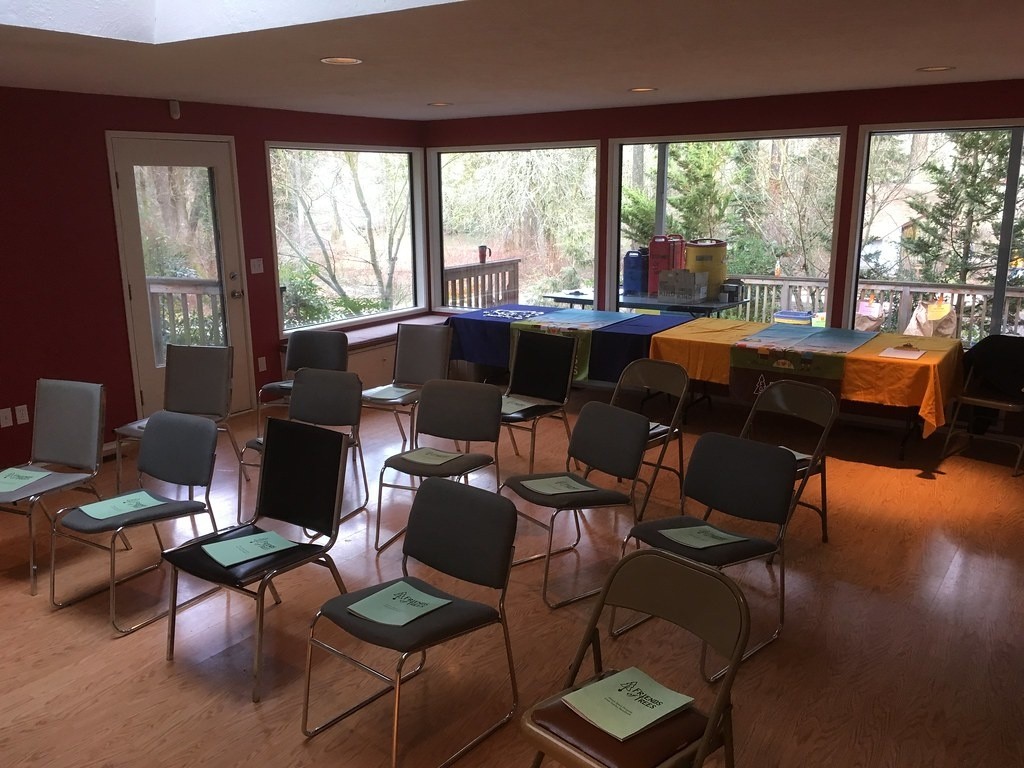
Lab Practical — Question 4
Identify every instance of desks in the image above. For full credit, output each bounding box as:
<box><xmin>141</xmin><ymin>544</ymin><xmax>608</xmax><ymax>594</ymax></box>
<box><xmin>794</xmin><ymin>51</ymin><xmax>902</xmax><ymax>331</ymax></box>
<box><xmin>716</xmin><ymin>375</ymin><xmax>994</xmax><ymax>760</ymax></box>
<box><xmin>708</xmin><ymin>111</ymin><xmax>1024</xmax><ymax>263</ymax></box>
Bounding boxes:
<box><xmin>545</xmin><ymin>286</ymin><xmax>751</xmax><ymax>318</ymax></box>
<box><xmin>447</xmin><ymin>303</ymin><xmax>963</xmax><ymax>479</ymax></box>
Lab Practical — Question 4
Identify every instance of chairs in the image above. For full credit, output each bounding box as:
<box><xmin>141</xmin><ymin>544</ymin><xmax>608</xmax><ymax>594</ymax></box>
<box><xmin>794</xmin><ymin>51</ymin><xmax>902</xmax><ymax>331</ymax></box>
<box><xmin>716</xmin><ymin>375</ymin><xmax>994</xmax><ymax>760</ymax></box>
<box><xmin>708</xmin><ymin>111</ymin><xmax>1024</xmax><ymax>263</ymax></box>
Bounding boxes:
<box><xmin>0</xmin><ymin>321</ymin><xmax>841</xmax><ymax>768</ymax></box>
<box><xmin>934</xmin><ymin>335</ymin><xmax>1024</xmax><ymax>477</ymax></box>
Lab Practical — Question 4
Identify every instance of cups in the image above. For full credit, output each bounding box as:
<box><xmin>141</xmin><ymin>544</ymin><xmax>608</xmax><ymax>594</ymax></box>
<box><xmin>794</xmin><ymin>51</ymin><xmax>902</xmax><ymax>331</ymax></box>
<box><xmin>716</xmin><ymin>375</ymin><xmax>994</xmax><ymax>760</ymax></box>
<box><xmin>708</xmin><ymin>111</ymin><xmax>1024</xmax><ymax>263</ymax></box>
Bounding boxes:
<box><xmin>479</xmin><ymin>246</ymin><xmax>491</xmax><ymax>263</ymax></box>
<box><xmin>623</xmin><ymin>247</ymin><xmax>648</xmax><ymax>297</ymax></box>
<box><xmin>718</xmin><ymin>293</ymin><xmax>728</xmax><ymax>302</ymax></box>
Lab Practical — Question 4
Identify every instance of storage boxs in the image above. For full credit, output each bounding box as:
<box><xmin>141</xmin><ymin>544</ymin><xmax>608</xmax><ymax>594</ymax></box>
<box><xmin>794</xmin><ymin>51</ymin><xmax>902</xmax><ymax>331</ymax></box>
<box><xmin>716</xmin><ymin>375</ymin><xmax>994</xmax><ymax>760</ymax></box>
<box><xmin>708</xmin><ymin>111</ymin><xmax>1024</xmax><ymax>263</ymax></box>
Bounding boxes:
<box><xmin>720</xmin><ymin>278</ymin><xmax>745</xmax><ymax>301</ymax></box>
<box><xmin>657</xmin><ymin>268</ymin><xmax>709</xmax><ymax>304</ymax></box>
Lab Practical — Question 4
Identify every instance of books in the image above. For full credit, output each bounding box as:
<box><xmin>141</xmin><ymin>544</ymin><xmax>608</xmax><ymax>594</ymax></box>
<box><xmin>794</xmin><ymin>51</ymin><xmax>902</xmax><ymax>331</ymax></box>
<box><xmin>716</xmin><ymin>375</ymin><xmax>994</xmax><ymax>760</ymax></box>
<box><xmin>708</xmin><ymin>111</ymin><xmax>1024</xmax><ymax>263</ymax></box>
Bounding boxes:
<box><xmin>77</xmin><ymin>490</ymin><xmax>168</xmax><ymax>521</ymax></box>
<box><xmin>366</xmin><ymin>386</ymin><xmax>416</xmax><ymax>400</ymax></box>
<box><xmin>561</xmin><ymin>666</ymin><xmax>695</xmax><ymax>741</ymax></box>
<box><xmin>657</xmin><ymin>525</ymin><xmax>750</xmax><ymax>548</ymax></box>
<box><xmin>0</xmin><ymin>467</ymin><xmax>53</xmax><ymax>493</ymax></box>
<box><xmin>401</xmin><ymin>447</ymin><xmax>463</xmax><ymax>466</ymax></box>
<box><xmin>346</xmin><ymin>580</ymin><xmax>452</xmax><ymax>627</ymax></box>
<box><xmin>519</xmin><ymin>475</ymin><xmax>599</xmax><ymax>496</ymax></box>
<box><xmin>200</xmin><ymin>530</ymin><xmax>299</xmax><ymax>568</ymax></box>
<box><xmin>501</xmin><ymin>396</ymin><xmax>538</xmax><ymax>415</ymax></box>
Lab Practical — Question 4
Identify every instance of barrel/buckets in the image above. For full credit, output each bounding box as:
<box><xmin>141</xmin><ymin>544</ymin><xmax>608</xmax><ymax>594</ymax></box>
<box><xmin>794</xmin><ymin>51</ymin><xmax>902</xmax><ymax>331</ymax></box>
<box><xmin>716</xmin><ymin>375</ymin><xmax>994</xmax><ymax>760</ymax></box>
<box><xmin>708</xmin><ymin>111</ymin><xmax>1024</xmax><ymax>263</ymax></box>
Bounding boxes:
<box><xmin>684</xmin><ymin>238</ymin><xmax>727</xmax><ymax>300</ymax></box>
<box><xmin>648</xmin><ymin>234</ymin><xmax>685</xmax><ymax>297</ymax></box>
